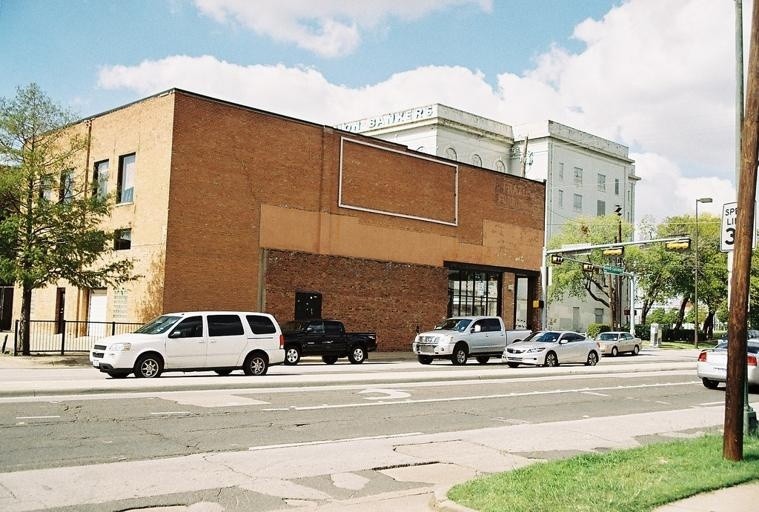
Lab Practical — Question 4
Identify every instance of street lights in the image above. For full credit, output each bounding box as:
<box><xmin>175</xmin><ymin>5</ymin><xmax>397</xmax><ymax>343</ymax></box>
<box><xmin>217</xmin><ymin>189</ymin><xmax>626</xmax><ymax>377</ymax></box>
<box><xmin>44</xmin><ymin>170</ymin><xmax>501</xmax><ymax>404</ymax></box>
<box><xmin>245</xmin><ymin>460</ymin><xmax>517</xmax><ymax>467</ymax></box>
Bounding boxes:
<box><xmin>695</xmin><ymin>197</ymin><xmax>713</xmax><ymax>348</ymax></box>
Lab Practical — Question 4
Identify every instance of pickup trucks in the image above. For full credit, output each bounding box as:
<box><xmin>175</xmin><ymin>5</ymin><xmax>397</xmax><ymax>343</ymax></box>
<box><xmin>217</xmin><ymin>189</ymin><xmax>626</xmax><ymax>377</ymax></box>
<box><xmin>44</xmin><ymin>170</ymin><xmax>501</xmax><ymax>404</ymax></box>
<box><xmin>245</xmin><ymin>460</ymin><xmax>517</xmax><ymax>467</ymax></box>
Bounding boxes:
<box><xmin>412</xmin><ymin>316</ymin><xmax>533</xmax><ymax>365</ymax></box>
<box><xmin>280</xmin><ymin>319</ymin><xmax>376</xmax><ymax>366</ymax></box>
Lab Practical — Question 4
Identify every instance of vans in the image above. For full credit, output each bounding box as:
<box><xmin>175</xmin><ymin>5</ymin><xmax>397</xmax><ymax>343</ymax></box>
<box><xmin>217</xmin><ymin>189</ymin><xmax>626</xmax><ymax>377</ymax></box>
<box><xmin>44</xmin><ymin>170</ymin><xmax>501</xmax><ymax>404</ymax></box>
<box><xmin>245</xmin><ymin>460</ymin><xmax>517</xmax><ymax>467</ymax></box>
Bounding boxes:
<box><xmin>90</xmin><ymin>312</ymin><xmax>286</xmax><ymax>378</ymax></box>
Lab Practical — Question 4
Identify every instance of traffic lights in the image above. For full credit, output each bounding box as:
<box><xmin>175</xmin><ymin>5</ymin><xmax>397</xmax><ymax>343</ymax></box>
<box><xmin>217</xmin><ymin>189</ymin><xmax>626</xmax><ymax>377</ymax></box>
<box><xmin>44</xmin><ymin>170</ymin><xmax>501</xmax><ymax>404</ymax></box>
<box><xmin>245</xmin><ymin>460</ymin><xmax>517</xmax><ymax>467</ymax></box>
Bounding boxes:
<box><xmin>602</xmin><ymin>247</ymin><xmax>624</xmax><ymax>257</ymax></box>
<box><xmin>581</xmin><ymin>263</ymin><xmax>595</xmax><ymax>272</ymax></box>
<box><xmin>665</xmin><ymin>239</ymin><xmax>691</xmax><ymax>253</ymax></box>
<box><xmin>550</xmin><ymin>255</ymin><xmax>564</xmax><ymax>265</ymax></box>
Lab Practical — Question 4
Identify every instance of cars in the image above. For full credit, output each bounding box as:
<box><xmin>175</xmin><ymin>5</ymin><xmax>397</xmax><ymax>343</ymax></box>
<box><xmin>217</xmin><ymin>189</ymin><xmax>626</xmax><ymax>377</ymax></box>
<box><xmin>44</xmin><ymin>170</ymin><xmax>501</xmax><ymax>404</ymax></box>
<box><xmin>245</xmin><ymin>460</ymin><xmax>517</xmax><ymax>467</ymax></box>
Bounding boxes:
<box><xmin>501</xmin><ymin>331</ymin><xmax>601</xmax><ymax>368</ymax></box>
<box><xmin>697</xmin><ymin>329</ymin><xmax>759</xmax><ymax>388</ymax></box>
<box><xmin>594</xmin><ymin>332</ymin><xmax>643</xmax><ymax>356</ymax></box>
